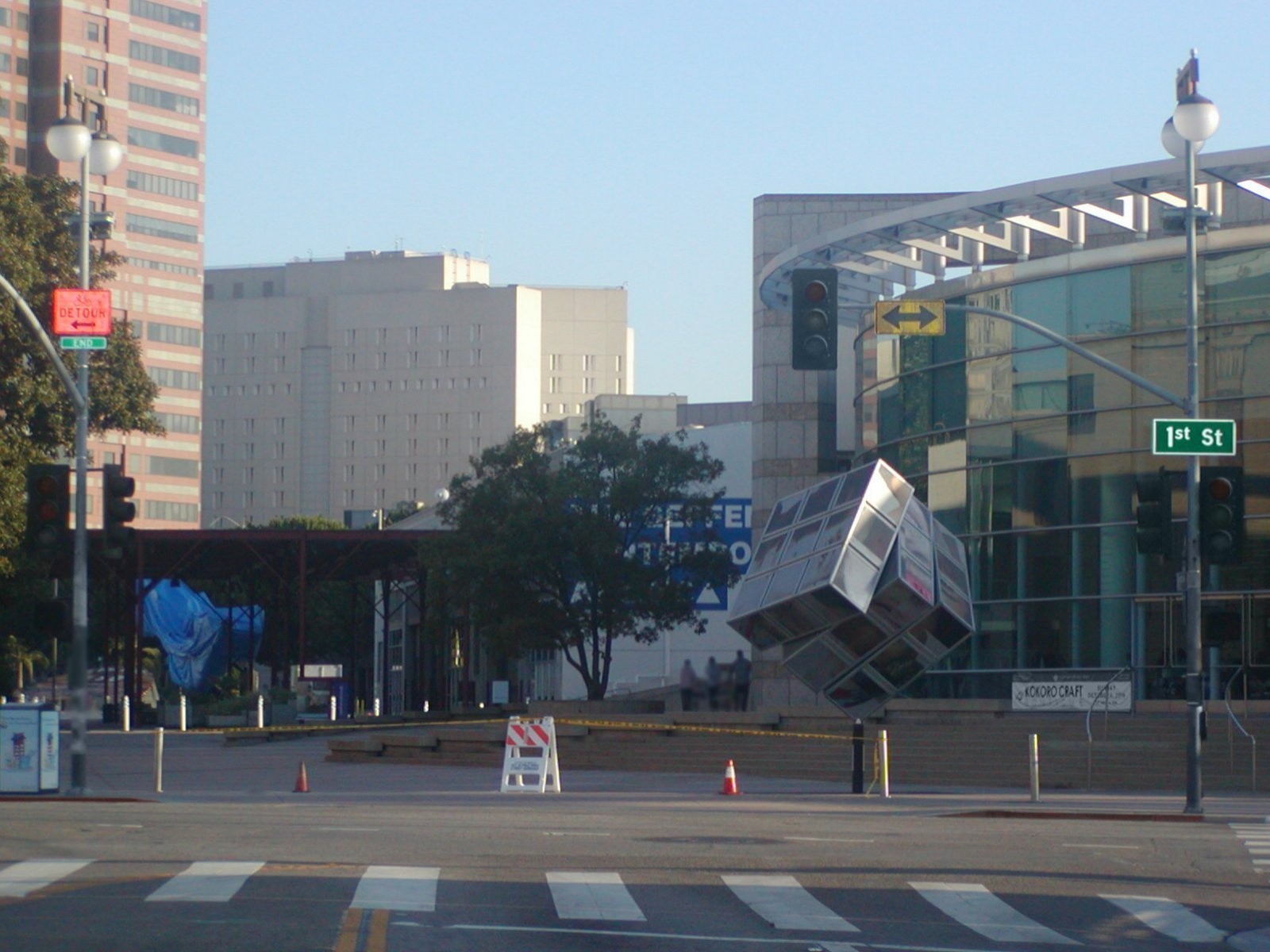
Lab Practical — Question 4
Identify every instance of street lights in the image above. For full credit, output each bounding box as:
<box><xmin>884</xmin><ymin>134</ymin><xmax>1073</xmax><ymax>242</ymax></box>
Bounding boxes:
<box><xmin>1159</xmin><ymin>46</ymin><xmax>1221</xmax><ymax>816</ymax></box>
<box><xmin>43</xmin><ymin>74</ymin><xmax>125</xmax><ymax>798</ymax></box>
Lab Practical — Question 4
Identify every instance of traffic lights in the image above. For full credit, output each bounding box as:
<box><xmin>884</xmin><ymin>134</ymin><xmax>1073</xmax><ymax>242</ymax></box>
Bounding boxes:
<box><xmin>1136</xmin><ymin>474</ymin><xmax>1173</xmax><ymax>561</ymax></box>
<box><xmin>1198</xmin><ymin>464</ymin><xmax>1246</xmax><ymax>560</ymax></box>
<box><xmin>103</xmin><ymin>462</ymin><xmax>136</xmax><ymax>561</ymax></box>
<box><xmin>792</xmin><ymin>267</ymin><xmax>839</xmax><ymax>371</ymax></box>
<box><xmin>25</xmin><ymin>463</ymin><xmax>71</xmax><ymax>563</ymax></box>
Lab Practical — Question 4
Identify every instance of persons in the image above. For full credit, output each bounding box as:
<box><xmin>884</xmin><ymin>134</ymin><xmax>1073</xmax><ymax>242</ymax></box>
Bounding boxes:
<box><xmin>680</xmin><ymin>659</ymin><xmax>696</xmax><ymax>711</ymax></box>
<box><xmin>728</xmin><ymin>649</ymin><xmax>751</xmax><ymax>711</ymax></box>
<box><xmin>703</xmin><ymin>656</ymin><xmax>720</xmax><ymax>710</ymax></box>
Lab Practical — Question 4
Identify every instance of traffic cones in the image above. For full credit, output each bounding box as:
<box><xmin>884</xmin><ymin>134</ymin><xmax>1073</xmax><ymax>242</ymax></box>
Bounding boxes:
<box><xmin>292</xmin><ymin>761</ymin><xmax>312</xmax><ymax>793</ymax></box>
<box><xmin>718</xmin><ymin>759</ymin><xmax>743</xmax><ymax>796</ymax></box>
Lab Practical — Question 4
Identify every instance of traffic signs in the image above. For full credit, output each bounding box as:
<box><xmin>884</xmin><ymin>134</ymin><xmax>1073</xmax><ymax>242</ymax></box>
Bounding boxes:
<box><xmin>51</xmin><ymin>290</ymin><xmax>113</xmax><ymax>338</ymax></box>
